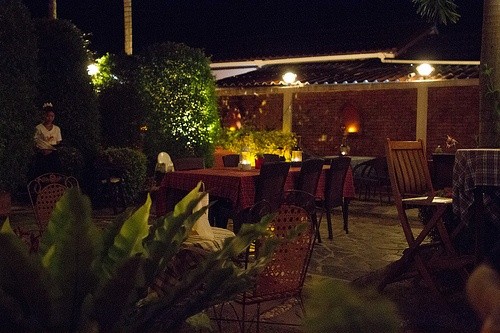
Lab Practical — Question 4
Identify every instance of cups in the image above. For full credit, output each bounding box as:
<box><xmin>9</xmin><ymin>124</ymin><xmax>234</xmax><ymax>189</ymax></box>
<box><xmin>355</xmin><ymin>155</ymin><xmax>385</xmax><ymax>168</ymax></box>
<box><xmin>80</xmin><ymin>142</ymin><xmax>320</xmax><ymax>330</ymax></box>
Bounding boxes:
<box><xmin>241</xmin><ymin>151</ymin><xmax>251</xmax><ymax>171</ymax></box>
<box><xmin>291</xmin><ymin>150</ymin><xmax>302</xmax><ymax>162</ymax></box>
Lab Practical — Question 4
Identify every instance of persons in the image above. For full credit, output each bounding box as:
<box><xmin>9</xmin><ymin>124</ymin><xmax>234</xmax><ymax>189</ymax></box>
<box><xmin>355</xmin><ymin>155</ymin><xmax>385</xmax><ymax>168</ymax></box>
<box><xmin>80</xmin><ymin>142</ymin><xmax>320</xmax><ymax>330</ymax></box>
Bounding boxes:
<box><xmin>32</xmin><ymin>103</ymin><xmax>62</xmax><ymax>179</ymax></box>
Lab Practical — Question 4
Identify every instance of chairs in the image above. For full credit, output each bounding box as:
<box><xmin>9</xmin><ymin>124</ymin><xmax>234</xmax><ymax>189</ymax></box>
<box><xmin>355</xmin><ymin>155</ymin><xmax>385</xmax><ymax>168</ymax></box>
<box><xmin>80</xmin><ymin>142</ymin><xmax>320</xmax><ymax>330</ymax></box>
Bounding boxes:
<box><xmin>153</xmin><ymin>152</ymin><xmax>392</xmax><ymax>333</ymax></box>
<box><xmin>27</xmin><ymin>171</ymin><xmax>80</xmax><ymax>240</ymax></box>
<box><xmin>377</xmin><ymin>138</ymin><xmax>460</xmax><ymax>301</ymax></box>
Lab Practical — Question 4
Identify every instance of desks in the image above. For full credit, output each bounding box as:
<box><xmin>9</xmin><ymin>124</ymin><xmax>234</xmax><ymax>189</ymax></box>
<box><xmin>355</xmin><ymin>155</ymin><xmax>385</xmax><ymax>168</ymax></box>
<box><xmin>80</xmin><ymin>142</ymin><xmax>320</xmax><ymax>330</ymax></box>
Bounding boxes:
<box><xmin>324</xmin><ymin>156</ymin><xmax>376</xmax><ymax>190</ymax></box>
<box><xmin>181</xmin><ymin>223</ymin><xmax>236</xmax><ymax>251</ymax></box>
<box><xmin>431</xmin><ymin>152</ymin><xmax>454</xmax><ymax>186</ymax></box>
<box><xmin>452</xmin><ymin>149</ymin><xmax>500</xmax><ymax>268</ymax></box>
<box><xmin>157</xmin><ymin>165</ymin><xmax>356</xmax><ymax>239</ymax></box>
